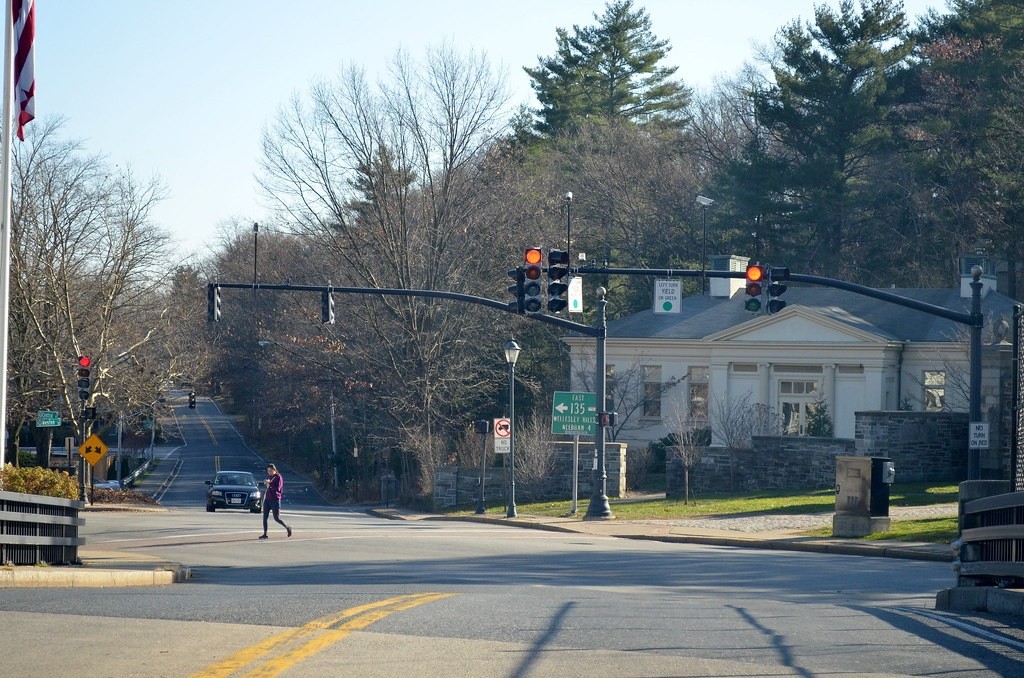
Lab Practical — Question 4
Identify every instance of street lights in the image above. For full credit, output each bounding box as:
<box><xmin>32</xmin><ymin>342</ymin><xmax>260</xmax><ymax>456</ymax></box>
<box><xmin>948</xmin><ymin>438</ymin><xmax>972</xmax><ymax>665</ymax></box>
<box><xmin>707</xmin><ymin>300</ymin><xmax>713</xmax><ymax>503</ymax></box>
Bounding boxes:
<box><xmin>502</xmin><ymin>335</ymin><xmax>521</xmax><ymax>518</ymax></box>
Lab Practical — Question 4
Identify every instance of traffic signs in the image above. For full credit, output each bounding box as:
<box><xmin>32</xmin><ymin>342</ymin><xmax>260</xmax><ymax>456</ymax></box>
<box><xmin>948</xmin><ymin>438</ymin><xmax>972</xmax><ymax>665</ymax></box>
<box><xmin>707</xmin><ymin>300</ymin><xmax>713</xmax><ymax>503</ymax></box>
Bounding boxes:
<box><xmin>552</xmin><ymin>391</ymin><xmax>596</xmax><ymax>435</ymax></box>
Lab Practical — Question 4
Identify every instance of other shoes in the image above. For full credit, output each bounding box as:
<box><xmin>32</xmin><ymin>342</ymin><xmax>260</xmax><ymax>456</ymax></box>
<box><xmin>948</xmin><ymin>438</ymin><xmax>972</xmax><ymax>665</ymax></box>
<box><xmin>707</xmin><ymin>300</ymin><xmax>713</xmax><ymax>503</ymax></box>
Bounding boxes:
<box><xmin>259</xmin><ymin>535</ymin><xmax>268</xmax><ymax>539</ymax></box>
<box><xmin>287</xmin><ymin>526</ymin><xmax>292</xmax><ymax>537</ymax></box>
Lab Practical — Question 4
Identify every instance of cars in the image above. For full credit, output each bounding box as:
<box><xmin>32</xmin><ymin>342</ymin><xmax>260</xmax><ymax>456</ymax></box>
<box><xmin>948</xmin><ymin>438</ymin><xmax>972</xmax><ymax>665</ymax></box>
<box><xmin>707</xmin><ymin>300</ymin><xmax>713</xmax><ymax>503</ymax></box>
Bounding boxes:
<box><xmin>205</xmin><ymin>471</ymin><xmax>266</xmax><ymax>514</ymax></box>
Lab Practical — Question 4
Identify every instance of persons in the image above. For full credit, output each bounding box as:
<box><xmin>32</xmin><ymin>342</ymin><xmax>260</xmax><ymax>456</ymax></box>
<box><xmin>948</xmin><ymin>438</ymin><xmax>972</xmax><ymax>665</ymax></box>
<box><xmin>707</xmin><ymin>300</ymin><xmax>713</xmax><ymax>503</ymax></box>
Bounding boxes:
<box><xmin>188</xmin><ymin>385</ymin><xmax>197</xmax><ymax>406</ymax></box>
<box><xmin>221</xmin><ymin>476</ymin><xmax>228</xmax><ymax>485</ymax></box>
<box><xmin>239</xmin><ymin>476</ymin><xmax>251</xmax><ymax>485</ymax></box>
<box><xmin>259</xmin><ymin>463</ymin><xmax>292</xmax><ymax>540</ymax></box>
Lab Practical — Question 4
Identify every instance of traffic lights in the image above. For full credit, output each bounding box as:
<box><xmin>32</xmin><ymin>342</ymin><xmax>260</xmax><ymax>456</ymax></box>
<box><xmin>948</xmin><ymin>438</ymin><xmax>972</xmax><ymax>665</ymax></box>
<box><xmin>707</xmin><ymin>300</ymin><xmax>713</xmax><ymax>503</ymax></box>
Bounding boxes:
<box><xmin>598</xmin><ymin>412</ymin><xmax>617</xmax><ymax>426</ymax></box>
<box><xmin>86</xmin><ymin>407</ymin><xmax>96</xmax><ymax>419</ymax></box>
<box><xmin>745</xmin><ymin>265</ymin><xmax>763</xmax><ymax>314</ymax></box>
<box><xmin>545</xmin><ymin>248</ymin><xmax>569</xmax><ymax>315</ymax></box>
<box><xmin>209</xmin><ymin>286</ymin><xmax>221</xmax><ymax>322</ymax></box>
<box><xmin>766</xmin><ymin>265</ymin><xmax>790</xmax><ymax>314</ymax></box>
<box><xmin>508</xmin><ymin>266</ymin><xmax>525</xmax><ymax>315</ymax></box>
<box><xmin>525</xmin><ymin>247</ymin><xmax>542</xmax><ymax>315</ymax></box>
<box><xmin>78</xmin><ymin>356</ymin><xmax>90</xmax><ymax>400</ymax></box>
